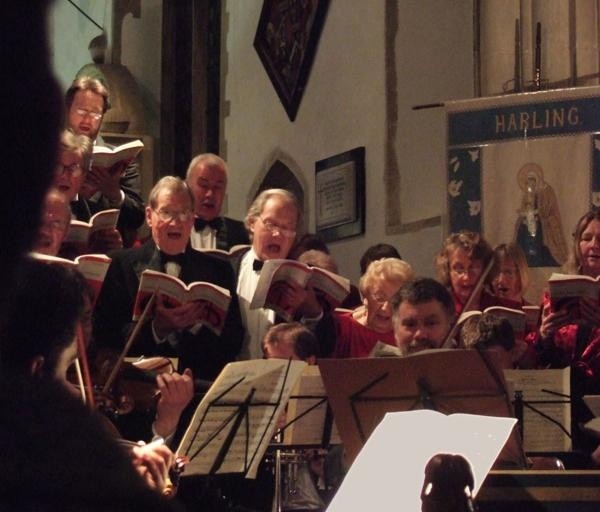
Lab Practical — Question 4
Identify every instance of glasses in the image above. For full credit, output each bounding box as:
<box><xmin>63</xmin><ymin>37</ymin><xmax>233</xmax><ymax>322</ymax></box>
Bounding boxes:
<box><xmin>55</xmin><ymin>161</ymin><xmax>84</xmax><ymax>179</ymax></box>
<box><xmin>72</xmin><ymin>106</ymin><xmax>103</xmax><ymax>121</ymax></box>
<box><xmin>153</xmin><ymin>210</ymin><xmax>193</xmax><ymax>222</ymax></box>
<box><xmin>261</xmin><ymin>216</ymin><xmax>297</xmax><ymax>239</ymax></box>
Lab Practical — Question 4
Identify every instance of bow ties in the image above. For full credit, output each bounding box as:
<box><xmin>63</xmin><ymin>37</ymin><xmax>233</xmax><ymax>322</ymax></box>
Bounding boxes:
<box><xmin>194</xmin><ymin>219</ymin><xmax>224</xmax><ymax>233</ymax></box>
<box><xmin>158</xmin><ymin>249</ymin><xmax>183</xmax><ymax>266</ymax></box>
<box><xmin>253</xmin><ymin>259</ymin><xmax>264</xmax><ymax>271</ymax></box>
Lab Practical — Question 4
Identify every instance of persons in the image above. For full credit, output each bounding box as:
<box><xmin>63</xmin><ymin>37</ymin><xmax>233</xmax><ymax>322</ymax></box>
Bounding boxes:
<box><xmin>24</xmin><ymin>77</ymin><xmax>599</xmax><ymax>512</ymax></box>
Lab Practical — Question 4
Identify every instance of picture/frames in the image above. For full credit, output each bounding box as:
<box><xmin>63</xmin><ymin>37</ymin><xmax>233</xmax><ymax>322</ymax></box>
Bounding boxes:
<box><xmin>253</xmin><ymin>0</ymin><xmax>331</xmax><ymax>123</ymax></box>
<box><xmin>314</xmin><ymin>145</ymin><xmax>366</xmax><ymax>244</ymax></box>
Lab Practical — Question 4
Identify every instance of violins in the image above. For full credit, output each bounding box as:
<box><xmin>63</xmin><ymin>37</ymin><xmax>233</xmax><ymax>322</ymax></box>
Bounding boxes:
<box><xmin>67</xmin><ymin>351</ymin><xmax>215</xmax><ymax>416</ymax></box>
